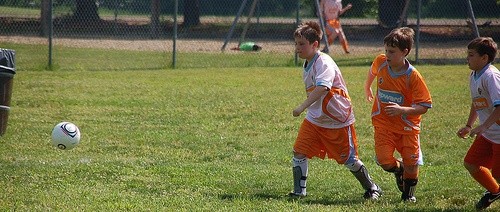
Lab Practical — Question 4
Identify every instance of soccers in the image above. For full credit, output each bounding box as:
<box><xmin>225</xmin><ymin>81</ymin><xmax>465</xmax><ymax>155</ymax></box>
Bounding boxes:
<box><xmin>50</xmin><ymin>122</ymin><xmax>81</xmax><ymax>149</ymax></box>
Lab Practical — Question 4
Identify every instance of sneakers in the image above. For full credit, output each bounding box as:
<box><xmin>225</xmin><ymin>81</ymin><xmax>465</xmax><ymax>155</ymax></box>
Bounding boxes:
<box><xmin>401</xmin><ymin>196</ymin><xmax>416</xmax><ymax>206</ymax></box>
<box><xmin>364</xmin><ymin>184</ymin><xmax>382</xmax><ymax>205</ymax></box>
<box><xmin>286</xmin><ymin>192</ymin><xmax>307</xmax><ymax>198</ymax></box>
<box><xmin>476</xmin><ymin>191</ymin><xmax>500</xmax><ymax>211</ymax></box>
<box><xmin>394</xmin><ymin>161</ymin><xmax>403</xmax><ymax>192</ymax></box>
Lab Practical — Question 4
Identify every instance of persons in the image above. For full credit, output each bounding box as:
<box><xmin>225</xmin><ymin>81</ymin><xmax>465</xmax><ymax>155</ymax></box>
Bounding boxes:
<box><xmin>365</xmin><ymin>27</ymin><xmax>432</xmax><ymax>203</ymax></box>
<box><xmin>456</xmin><ymin>37</ymin><xmax>500</xmax><ymax>208</ymax></box>
<box><xmin>317</xmin><ymin>0</ymin><xmax>352</xmax><ymax>54</ymax></box>
<box><xmin>230</xmin><ymin>41</ymin><xmax>262</xmax><ymax>52</ymax></box>
<box><xmin>286</xmin><ymin>23</ymin><xmax>383</xmax><ymax>202</ymax></box>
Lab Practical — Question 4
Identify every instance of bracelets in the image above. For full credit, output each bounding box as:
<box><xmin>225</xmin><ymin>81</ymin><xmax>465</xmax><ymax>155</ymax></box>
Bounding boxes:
<box><xmin>466</xmin><ymin>124</ymin><xmax>472</xmax><ymax>128</ymax></box>
